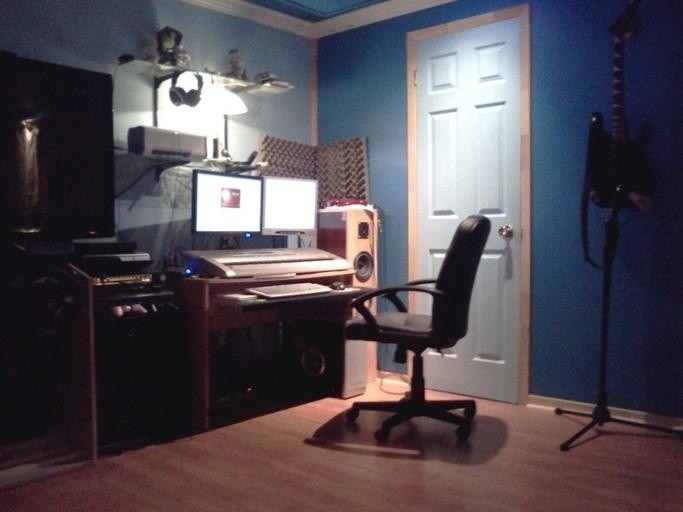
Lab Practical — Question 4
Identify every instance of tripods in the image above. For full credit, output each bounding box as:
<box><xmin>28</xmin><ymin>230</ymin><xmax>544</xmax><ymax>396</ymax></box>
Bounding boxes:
<box><xmin>555</xmin><ymin>209</ymin><xmax>683</xmax><ymax>451</ymax></box>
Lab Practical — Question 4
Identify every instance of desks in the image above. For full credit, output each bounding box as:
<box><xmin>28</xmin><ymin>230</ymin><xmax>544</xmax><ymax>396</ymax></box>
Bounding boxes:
<box><xmin>57</xmin><ymin>245</ymin><xmax>358</xmax><ymax>458</ymax></box>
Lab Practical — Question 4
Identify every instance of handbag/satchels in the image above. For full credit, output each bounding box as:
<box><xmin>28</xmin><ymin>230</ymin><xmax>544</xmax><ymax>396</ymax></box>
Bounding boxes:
<box><xmin>590</xmin><ymin>133</ymin><xmax>653</xmax><ymax>207</ymax></box>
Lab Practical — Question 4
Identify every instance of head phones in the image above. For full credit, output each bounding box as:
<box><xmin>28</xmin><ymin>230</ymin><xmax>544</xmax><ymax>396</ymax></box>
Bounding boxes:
<box><xmin>170</xmin><ymin>69</ymin><xmax>202</xmax><ymax>105</ymax></box>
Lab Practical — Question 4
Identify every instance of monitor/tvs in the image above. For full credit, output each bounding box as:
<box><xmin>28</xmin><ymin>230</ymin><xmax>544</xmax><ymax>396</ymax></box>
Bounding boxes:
<box><xmin>191</xmin><ymin>169</ymin><xmax>263</xmax><ymax>234</ymax></box>
<box><xmin>263</xmin><ymin>176</ymin><xmax>319</xmax><ymax>236</ymax></box>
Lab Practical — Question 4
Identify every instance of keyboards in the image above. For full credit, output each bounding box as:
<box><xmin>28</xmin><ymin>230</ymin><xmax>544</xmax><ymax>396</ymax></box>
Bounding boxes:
<box><xmin>246</xmin><ymin>283</ymin><xmax>331</xmax><ymax>298</ymax></box>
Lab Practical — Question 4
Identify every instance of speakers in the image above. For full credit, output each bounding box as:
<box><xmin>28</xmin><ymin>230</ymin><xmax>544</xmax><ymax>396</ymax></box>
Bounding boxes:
<box><xmin>281</xmin><ymin>211</ymin><xmax>378</xmax><ymax>399</ymax></box>
<box><xmin>94</xmin><ymin>292</ymin><xmax>195</xmax><ymax>456</ymax></box>
<box><xmin>127</xmin><ymin>126</ymin><xmax>207</xmax><ymax>160</ymax></box>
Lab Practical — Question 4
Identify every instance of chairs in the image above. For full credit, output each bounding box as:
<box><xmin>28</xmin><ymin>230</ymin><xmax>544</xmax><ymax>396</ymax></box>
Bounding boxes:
<box><xmin>341</xmin><ymin>216</ymin><xmax>491</xmax><ymax>444</ymax></box>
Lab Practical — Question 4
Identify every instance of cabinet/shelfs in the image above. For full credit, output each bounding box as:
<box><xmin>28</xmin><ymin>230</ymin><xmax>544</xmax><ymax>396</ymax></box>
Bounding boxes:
<box><xmin>113</xmin><ymin>58</ymin><xmax>268</xmax><ymax>172</ymax></box>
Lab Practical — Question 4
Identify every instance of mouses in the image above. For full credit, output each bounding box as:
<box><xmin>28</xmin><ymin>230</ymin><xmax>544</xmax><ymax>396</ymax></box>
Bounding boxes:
<box><xmin>331</xmin><ymin>282</ymin><xmax>345</xmax><ymax>290</ymax></box>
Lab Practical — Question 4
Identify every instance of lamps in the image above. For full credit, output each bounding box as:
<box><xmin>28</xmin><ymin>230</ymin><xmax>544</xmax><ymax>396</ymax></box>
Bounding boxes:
<box><xmin>152</xmin><ymin>70</ymin><xmax>247</xmax><ymax>127</ymax></box>
